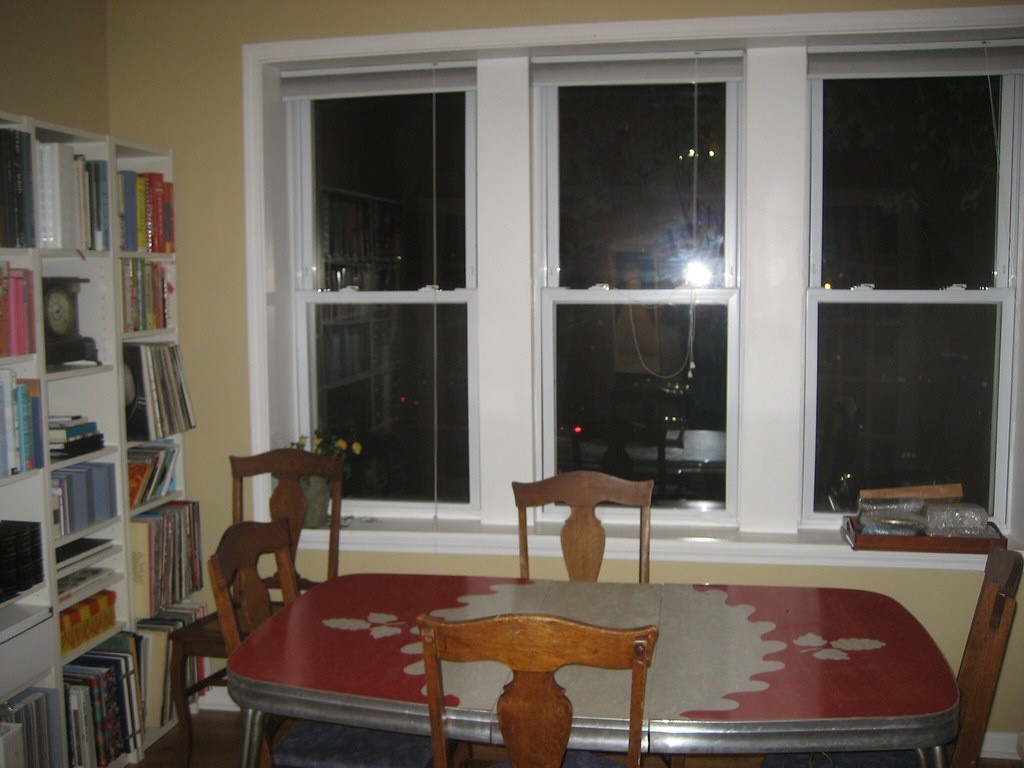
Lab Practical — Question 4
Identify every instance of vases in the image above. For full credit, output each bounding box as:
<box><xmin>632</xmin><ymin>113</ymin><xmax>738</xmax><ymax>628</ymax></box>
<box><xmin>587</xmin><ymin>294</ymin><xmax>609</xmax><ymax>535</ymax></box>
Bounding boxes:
<box><xmin>275</xmin><ymin>473</ymin><xmax>333</xmax><ymax>529</ymax></box>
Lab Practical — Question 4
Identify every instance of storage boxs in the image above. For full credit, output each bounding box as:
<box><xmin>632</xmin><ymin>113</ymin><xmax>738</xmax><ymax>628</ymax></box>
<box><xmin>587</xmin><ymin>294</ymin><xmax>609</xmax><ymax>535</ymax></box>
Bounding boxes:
<box><xmin>842</xmin><ymin>512</ymin><xmax>1009</xmax><ymax>554</ymax></box>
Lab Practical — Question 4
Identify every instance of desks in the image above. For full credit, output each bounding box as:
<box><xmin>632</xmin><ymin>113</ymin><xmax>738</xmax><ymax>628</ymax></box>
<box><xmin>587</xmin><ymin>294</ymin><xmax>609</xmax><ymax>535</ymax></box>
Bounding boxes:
<box><xmin>225</xmin><ymin>557</ymin><xmax>964</xmax><ymax>768</ymax></box>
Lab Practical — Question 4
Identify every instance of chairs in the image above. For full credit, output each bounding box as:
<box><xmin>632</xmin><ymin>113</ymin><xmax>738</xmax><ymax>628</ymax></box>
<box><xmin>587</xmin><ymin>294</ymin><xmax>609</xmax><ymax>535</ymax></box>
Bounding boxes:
<box><xmin>759</xmin><ymin>547</ymin><xmax>1023</xmax><ymax>768</ymax></box>
<box><xmin>513</xmin><ymin>469</ymin><xmax>653</xmax><ymax>585</ymax></box>
<box><xmin>206</xmin><ymin>516</ymin><xmax>464</xmax><ymax>766</ymax></box>
<box><xmin>165</xmin><ymin>448</ymin><xmax>341</xmax><ymax>768</ymax></box>
<box><xmin>418</xmin><ymin>612</ymin><xmax>669</xmax><ymax>768</ymax></box>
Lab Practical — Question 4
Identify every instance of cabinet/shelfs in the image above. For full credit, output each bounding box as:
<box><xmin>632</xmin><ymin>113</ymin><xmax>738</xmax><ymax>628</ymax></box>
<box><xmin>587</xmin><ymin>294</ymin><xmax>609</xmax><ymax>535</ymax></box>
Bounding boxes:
<box><xmin>309</xmin><ymin>183</ymin><xmax>431</xmax><ymax>497</ymax></box>
<box><xmin>0</xmin><ymin>111</ymin><xmax>211</xmax><ymax>768</ymax></box>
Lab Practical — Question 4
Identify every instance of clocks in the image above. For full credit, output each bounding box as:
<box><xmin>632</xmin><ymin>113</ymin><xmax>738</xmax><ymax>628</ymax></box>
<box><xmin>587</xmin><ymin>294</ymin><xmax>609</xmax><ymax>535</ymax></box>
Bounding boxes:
<box><xmin>41</xmin><ymin>287</ymin><xmax>73</xmax><ymax>337</ymax></box>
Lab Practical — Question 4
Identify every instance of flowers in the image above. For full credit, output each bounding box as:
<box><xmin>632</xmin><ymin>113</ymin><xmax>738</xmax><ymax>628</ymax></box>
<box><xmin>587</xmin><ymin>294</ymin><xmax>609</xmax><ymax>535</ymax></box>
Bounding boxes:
<box><xmin>287</xmin><ymin>434</ymin><xmax>363</xmax><ymax>480</ymax></box>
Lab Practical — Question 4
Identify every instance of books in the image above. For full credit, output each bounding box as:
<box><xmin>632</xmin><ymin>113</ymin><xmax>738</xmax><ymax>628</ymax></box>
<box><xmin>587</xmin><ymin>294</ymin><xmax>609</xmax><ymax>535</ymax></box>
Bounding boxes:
<box><xmin>315</xmin><ymin>192</ymin><xmax>423</xmax><ymax>503</ymax></box>
<box><xmin>116</xmin><ymin>170</ymin><xmax>174</xmax><ymax>253</ymax></box>
<box><xmin>136</xmin><ymin>603</ymin><xmax>213</xmax><ymax>729</ymax></box>
<box><xmin>131</xmin><ymin>501</ymin><xmax>203</xmax><ymax>619</ymax></box>
<box><xmin>0</xmin><ymin>126</ymin><xmax>33</xmax><ymax>250</ymax></box>
<box><xmin>1</xmin><ymin>519</ymin><xmax>45</xmax><ymax>602</ymax></box>
<box><xmin>0</xmin><ymin>259</ymin><xmax>37</xmax><ymax>357</ymax></box>
<box><xmin>59</xmin><ymin>565</ymin><xmax>126</xmax><ymax>614</ymax></box>
<box><xmin>119</xmin><ymin>339</ymin><xmax>196</xmax><ymax>442</ymax></box>
<box><xmin>0</xmin><ymin>687</ymin><xmax>63</xmax><ymax>768</ymax></box>
<box><xmin>51</xmin><ymin>461</ymin><xmax>116</xmax><ymax>538</ymax></box>
<box><xmin>126</xmin><ymin>439</ymin><xmax>181</xmax><ymax>509</ymax></box>
<box><xmin>0</xmin><ymin>366</ymin><xmax>41</xmax><ymax>475</ymax></box>
<box><xmin>35</xmin><ymin>142</ymin><xmax>108</xmax><ymax>251</ymax></box>
<box><xmin>45</xmin><ymin>415</ymin><xmax>105</xmax><ymax>461</ymax></box>
<box><xmin>62</xmin><ymin>630</ymin><xmax>146</xmax><ymax>768</ymax></box>
<box><xmin>121</xmin><ymin>256</ymin><xmax>170</xmax><ymax>333</ymax></box>
